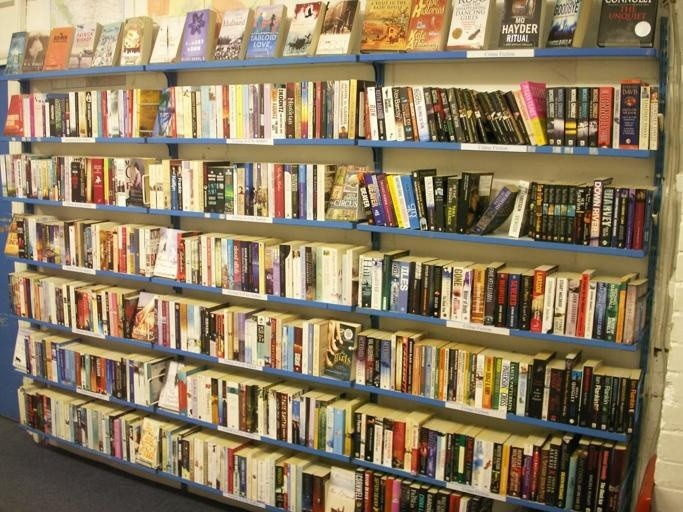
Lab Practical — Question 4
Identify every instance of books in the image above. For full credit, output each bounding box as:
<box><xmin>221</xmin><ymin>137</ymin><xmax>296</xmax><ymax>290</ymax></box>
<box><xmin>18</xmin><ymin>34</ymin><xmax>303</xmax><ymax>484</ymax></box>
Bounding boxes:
<box><xmin>179</xmin><ymin>5</ymin><xmax>287</xmax><ymax>63</ymax></box>
<box><xmin>449</xmin><ymin>0</ymin><xmax>662</xmax><ymax>48</ymax></box>
<box><xmin>2</xmin><ymin>28</ymin><xmax>76</xmax><ymax>72</ymax></box>
<box><xmin>283</xmin><ymin>1</ymin><xmax>446</xmax><ymax>57</ymax></box>
<box><xmin>3</xmin><ymin>78</ymin><xmax>662</xmax><ymax>153</ymax></box>
<box><xmin>3</xmin><ymin>216</ymin><xmax>642</xmax><ymax>510</ymax></box>
<box><xmin>4</xmin><ymin>150</ymin><xmax>656</xmax><ymax>251</ymax></box>
<box><xmin>69</xmin><ymin>14</ymin><xmax>187</xmax><ymax>68</ymax></box>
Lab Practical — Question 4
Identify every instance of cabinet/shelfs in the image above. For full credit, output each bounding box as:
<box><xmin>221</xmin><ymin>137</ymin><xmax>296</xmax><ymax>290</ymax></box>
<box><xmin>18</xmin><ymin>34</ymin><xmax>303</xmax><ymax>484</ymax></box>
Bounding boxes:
<box><xmin>0</xmin><ymin>19</ymin><xmax>679</xmax><ymax>512</ymax></box>
